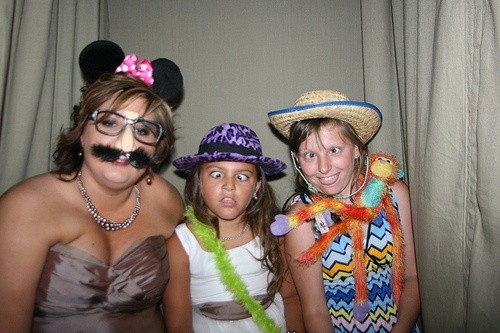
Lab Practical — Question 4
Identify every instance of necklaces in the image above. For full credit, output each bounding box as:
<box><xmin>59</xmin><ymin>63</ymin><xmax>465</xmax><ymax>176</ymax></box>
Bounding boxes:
<box><xmin>220</xmin><ymin>222</ymin><xmax>250</xmax><ymax>240</ymax></box>
<box><xmin>77</xmin><ymin>172</ymin><xmax>141</xmax><ymax>231</ymax></box>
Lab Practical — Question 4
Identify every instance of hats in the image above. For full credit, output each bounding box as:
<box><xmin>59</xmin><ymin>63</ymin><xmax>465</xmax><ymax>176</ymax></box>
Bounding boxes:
<box><xmin>267</xmin><ymin>90</ymin><xmax>384</xmax><ymax>147</ymax></box>
<box><xmin>172</xmin><ymin>122</ymin><xmax>288</xmax><ymax>175</ymax></box>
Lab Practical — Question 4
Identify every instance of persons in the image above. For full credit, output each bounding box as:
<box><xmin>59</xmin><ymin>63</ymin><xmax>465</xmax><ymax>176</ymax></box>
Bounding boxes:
<box><xmin>0</xmin><ymin>40</ymin><xmax>186</xmax><ymax>333</ymax></box>
<box><xmin>164</xmin><ymin>123</ymin><xmax>305</xmax><ymax>333</ymax></box>
<box><xmin>267</xmin><ymin>89</ymin><xmax>421</xmax><ymax>333</ymax></box>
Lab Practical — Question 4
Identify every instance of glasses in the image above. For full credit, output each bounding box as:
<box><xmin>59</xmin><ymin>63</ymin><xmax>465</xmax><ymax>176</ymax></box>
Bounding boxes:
<box><xmin>87</xmin><ymin>109</ymin><xmax>169</xmax><ymax>147</ymax></box>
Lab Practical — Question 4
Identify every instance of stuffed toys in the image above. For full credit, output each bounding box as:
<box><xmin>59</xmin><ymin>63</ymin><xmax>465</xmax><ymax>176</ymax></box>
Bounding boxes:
<box><xmin>270</xmin><ymin>151</ymin><xmax>404</xmax><ymax>306</ymax></box>
